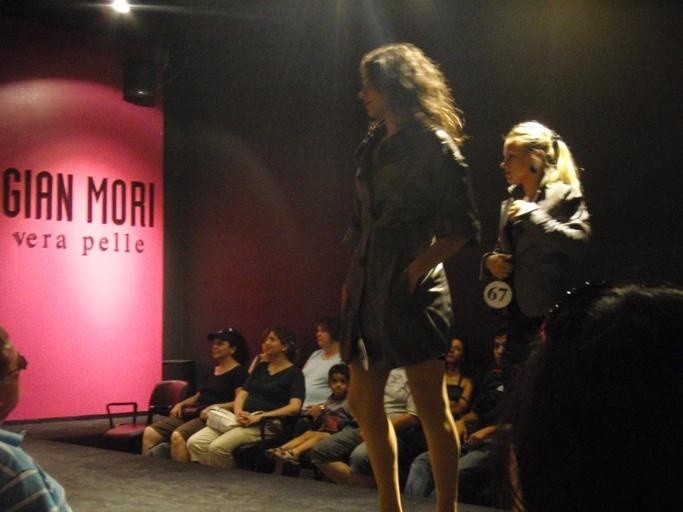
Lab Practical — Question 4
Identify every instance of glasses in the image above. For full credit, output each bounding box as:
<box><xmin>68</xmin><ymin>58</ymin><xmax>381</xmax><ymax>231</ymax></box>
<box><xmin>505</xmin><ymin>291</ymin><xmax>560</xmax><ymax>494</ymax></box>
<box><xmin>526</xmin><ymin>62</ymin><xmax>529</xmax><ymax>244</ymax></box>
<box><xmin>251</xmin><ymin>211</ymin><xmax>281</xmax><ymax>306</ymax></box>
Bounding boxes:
<box><xmin>10</xmin><ymin>356</ymin><xmax>27</xmax><ymax>373</ymax></box>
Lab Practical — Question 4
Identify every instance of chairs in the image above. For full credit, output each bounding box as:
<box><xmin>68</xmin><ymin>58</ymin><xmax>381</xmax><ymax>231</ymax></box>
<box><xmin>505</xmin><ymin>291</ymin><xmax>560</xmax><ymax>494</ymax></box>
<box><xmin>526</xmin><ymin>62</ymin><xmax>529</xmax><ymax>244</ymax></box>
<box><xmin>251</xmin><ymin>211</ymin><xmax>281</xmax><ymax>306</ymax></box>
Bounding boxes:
<box><xmin>106</xmin><ymin>380</ymin><xmax>314</xmax><ymax>472</ymax></box>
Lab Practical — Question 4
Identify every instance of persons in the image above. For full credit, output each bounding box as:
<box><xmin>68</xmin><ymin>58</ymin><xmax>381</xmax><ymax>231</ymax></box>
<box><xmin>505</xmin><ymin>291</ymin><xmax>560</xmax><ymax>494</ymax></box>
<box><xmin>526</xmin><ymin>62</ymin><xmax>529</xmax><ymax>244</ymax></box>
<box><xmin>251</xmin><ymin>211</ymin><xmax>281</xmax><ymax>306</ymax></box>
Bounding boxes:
<box><xmin>509</xmin><ymin>280</ymin><xmax>683</xmax><ymax>512</ymax></box>
<box><xmin>480</xmin><ymin>120</ymin><xmax>593</xmax><ymax>332</ymax></box>
<box><xmin>340</xmin><ymin>40</ymin><xmax>480</xmax><ymax>512</ymax></box>
<box><xmin>140</xmin><ymin>324</ymin><xmax>518</xmax><ymax>503</ymax></box>
<box><xmin>0</xmin><ymin>323</ymin><xmax>73</xmax><ymax>511</ymax></box>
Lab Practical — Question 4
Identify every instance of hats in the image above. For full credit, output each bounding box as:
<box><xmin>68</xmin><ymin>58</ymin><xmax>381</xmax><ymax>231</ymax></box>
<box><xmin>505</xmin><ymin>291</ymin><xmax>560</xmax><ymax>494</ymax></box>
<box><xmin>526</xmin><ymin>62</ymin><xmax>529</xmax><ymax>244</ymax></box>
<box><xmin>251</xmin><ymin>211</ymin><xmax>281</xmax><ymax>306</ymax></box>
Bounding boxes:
<box><xmin>208</xmin><ymin>328</ymin><xmax>239</xmax><ymax>341</ymax></box>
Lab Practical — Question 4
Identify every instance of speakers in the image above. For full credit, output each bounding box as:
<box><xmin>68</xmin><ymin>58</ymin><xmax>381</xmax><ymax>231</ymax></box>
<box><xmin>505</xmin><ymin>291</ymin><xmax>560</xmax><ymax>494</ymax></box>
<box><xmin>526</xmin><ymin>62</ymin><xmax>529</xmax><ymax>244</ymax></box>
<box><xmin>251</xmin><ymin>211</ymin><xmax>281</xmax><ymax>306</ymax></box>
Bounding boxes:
<box><xmin>125</xmin><ymin>57</ymin><xmax>156</xmax><ymax>100</ymax></box>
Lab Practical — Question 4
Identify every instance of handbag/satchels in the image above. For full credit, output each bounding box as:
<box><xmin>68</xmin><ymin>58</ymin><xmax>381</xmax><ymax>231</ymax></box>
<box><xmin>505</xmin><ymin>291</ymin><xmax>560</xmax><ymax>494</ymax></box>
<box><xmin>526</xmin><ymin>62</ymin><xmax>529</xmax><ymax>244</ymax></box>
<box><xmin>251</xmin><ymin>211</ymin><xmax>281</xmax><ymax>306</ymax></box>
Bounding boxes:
<box><xmin>205</xmin><ymin>407</ymin><xmax>242</xmax><ymax>433</ymax></box>
<box><xmin>312</xmin><ymin>408</ymin><xmax>353</xmax><ymax>433</ymax></box>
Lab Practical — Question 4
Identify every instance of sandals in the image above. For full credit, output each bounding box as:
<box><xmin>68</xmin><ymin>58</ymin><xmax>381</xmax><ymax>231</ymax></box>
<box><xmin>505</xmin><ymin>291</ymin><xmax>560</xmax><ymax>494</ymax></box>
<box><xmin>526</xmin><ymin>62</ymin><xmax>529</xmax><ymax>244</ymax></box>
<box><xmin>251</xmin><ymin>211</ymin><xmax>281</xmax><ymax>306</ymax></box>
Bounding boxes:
<box><xmin>267</xmin><ymin>447</ymin><xmax>300</xmax><ymax>465</ymax></box>
